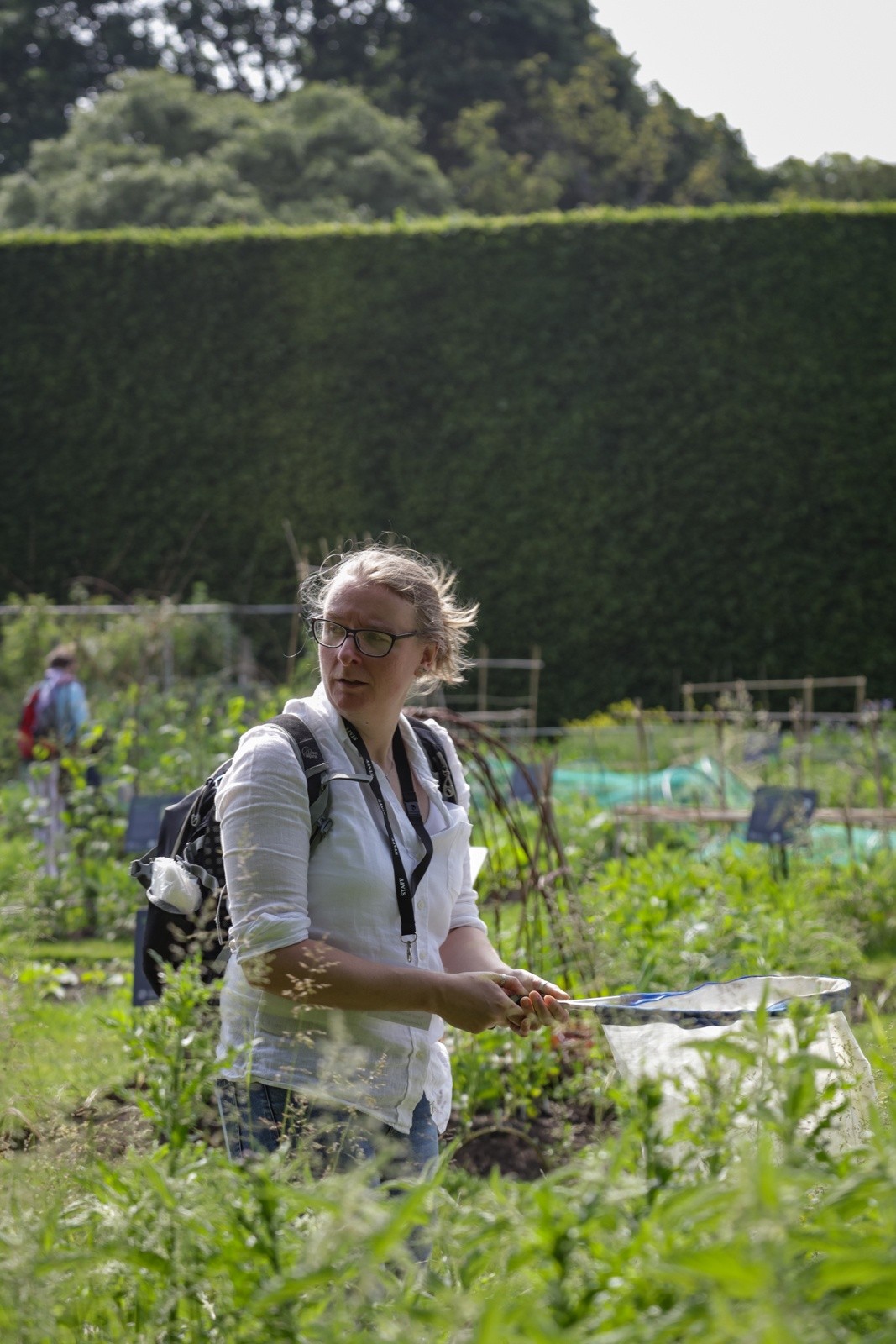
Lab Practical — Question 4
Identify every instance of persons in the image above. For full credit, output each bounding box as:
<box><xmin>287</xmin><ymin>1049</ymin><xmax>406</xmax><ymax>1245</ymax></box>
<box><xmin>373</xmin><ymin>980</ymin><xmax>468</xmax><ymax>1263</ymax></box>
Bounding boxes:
<box><xmin>13</xmin><ymin>637</ymin><xmax>103</xmax><ymax>891</ymax></box>
<box><xmin>128</xmin><ymin>544</ymin><xmax>571</xmax><ymax>1274</ymax></box>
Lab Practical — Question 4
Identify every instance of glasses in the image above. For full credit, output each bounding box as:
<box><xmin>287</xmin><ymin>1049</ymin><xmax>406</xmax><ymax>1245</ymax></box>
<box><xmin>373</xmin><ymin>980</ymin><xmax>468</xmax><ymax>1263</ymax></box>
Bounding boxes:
<box><xmin>311</xmin><ymin>613</ymin><xmax>428</xmax><ymax>658</ymax></box>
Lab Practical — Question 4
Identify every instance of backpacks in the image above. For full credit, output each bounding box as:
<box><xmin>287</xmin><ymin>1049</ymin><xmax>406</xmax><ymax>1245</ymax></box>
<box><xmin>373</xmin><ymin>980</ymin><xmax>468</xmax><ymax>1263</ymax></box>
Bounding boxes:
<box><xmin>16</xmin><ymin>678</ymin><xmax>72</xmax><ymax>762</ymax></box>
<box><xmin>127</xmin><ymin>713</ymin><xmax>458</xmax><ymax>1006</ymax></box>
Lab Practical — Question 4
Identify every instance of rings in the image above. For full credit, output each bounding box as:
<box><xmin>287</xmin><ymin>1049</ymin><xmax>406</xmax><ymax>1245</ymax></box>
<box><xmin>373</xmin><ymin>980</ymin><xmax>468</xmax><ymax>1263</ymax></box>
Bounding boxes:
<box><xmin>500</xmin><ymin>974</ymin><xmax>505</xmax><ymax>988</ymax></box>
<box><xmin>539</xmin><ymin>980</ymin><xmax>548</xmax><ymax>990</ymax></box>
